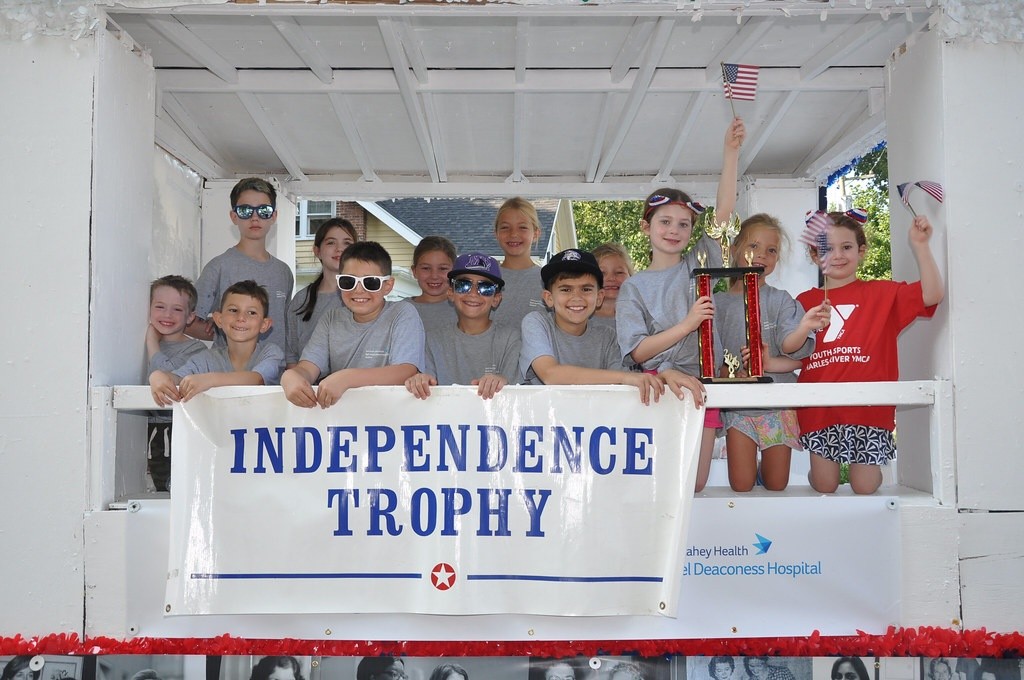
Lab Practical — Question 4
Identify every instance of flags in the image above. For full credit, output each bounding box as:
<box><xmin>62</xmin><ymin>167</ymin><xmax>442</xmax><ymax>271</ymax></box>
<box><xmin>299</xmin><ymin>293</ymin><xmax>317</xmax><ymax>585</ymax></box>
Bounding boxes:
<box><xmin>722</xmin><ymin>63</ymin><xmax>759</xmax><ymax>102</ymax></box>
<box><xmin>898</xmin><ymin>180</ymin><xmax>943</xmax><ymax>204</ymax></box>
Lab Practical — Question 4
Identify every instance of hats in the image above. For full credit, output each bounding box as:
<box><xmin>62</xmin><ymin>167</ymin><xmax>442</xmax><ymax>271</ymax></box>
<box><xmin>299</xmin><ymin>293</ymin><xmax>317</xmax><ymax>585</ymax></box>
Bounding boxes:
<box><xmin>447</xmin><ymin>251</ymin><xmax>505</xmax><ymax>287</ymax></box>
<box><xmin>541</xmin><ymin>248</ymin><xmax>604</xmax><ymax>286</ymax></box>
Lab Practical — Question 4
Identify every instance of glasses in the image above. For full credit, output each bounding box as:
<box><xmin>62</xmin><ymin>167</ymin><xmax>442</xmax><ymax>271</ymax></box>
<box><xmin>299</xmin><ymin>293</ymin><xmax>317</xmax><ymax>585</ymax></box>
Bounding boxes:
<box><xmin>336</xmin><ymin>275</ymin><xmax>390</xmax><ymax>292</ymax></box>
<box><xmin>234</xmin><ymin>204</ymin><xmax>275</xmax><ymax>219</ymax></box>
<box><xmin>450</xmin><ymin>280</ymin><xmax>498</xmax><ymax>297</ymax></box>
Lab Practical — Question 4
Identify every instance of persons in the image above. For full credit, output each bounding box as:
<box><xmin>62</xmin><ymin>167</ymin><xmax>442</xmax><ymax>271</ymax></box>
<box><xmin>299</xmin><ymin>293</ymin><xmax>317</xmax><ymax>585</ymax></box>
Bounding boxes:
<box><xmin>0</xmin><ymin>651</ymin><xmax>1021</xmax><ymax>680</ymax></box>
<box><xmin>146</xmin><ymin>115</ymin><xmax>945</xmax><ymax>494</ymax></box>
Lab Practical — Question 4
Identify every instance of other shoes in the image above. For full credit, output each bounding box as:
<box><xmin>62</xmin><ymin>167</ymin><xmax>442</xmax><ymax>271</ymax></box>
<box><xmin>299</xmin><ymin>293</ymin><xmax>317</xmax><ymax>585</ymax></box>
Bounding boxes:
<box><xmin>754</xmin><ymin>459</ymin><xmax>763</xmax><ymax>486</ymax></box>
